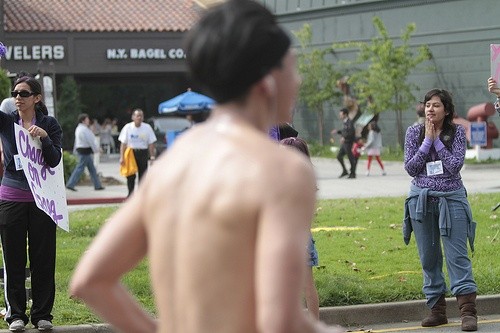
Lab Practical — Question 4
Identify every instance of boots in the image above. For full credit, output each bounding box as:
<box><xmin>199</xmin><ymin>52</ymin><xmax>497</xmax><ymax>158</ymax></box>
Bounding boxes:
<box><xmin>456</xmin><ymin>292</ymin><xmax>478</xmax><ymax>330</ymax></box>
<box><xmin>421</xmin><ymin>294</ymin><xmax>448</xmax><ymax>326</ymax></box>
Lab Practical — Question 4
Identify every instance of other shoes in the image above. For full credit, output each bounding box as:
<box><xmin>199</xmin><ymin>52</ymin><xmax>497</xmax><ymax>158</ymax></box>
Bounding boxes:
<box><xmin>338</xmin><ymin>171</ymin><xmax>348</xmax><ymax>177</ymax></box>
<box><xmin>348</xmin><ymin>173</ymin><xmax>355</xmax><ymax>178</ymax></box>
<box><xmin>367</xmin><ymin>171</ymin><xmax>371</xmax><ymax>176</ymax></box>
<box><xmin>66</xmin><ymin>186</ymin><xmax>77</xmax><ymax>191</ymax></box>
<box><xmin>381</xmin><ymin>172</ymin><xmax>388</xmax><ymax>176</ymax></box>
<box><xmin>95</xmin><ymin>186</ymin><xmax>104</xmax><ymax>190</ymax></box>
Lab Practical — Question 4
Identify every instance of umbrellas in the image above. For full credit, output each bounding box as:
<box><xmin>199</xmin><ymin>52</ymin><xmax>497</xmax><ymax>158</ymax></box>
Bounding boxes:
<box><xmin>158</xmin><ymin>87</ymin><xmax>215</xmax><ymax>123</ymax></box>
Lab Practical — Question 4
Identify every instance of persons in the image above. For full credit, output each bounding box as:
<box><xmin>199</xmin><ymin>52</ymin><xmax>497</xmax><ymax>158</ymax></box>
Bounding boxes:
<box><xmin>70</xmin><ymin>0</ymin><xmax>347</xmax><ymax>333</ymax></box>
<box><xmin>488</xmin><ymin>78</ymin><xmax>500</xmax><ymax>115</ymax></box>
<box><xmin>404</xmin><ymin>89</ymin><xmax>478</xmax><ymax>331</ymax></box>
<box><xmin>269</xmin><ymin>102</ymin><xmax>427</xmax><ymax>322</ymax></box>
<box><xmin>0</xmin><ymin>78</ymin><xmax>195</xmax><ymax>333</ymax></box>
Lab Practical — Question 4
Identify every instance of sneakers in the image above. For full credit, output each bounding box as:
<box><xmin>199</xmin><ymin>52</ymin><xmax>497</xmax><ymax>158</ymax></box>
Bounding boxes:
<box><xmin>36</xmin><ymin>320</ymin><xmax>54</xmax><ymax>330</ymax></box>
<box><xmin>9</xmin><ymin>320</ymin><xmax>25</xmax><ymax>331</ymax></box>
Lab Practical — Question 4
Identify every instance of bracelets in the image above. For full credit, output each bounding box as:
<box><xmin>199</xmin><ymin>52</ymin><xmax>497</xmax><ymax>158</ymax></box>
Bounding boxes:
<box><xmin>150</xmin><ymin>156</ymin><xmax>155</xmax><ymax>159</ymax></box>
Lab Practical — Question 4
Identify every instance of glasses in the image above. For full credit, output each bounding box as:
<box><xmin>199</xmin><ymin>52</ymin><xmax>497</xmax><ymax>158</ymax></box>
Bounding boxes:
<box><xmin>10</xmin><ymin>89</ymin><xmax>41</xmax><ymax>98</ymax></box>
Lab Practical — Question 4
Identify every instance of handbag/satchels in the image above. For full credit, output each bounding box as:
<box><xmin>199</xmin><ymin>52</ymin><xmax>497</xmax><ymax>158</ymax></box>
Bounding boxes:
<box><xmin>120</xmin><ymin>147</ymin><xmax>138</xmax><ymax>177</ymax></box>
